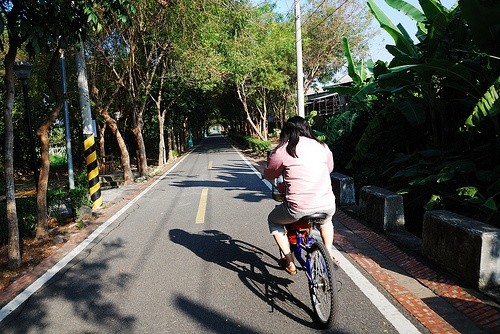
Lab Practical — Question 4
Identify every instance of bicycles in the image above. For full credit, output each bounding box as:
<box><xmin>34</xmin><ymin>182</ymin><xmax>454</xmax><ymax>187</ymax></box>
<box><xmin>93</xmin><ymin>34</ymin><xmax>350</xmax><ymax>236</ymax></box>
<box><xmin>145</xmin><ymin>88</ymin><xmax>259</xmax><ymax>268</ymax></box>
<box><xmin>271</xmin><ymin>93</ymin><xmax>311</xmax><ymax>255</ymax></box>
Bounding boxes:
<box><xmin>263</xmin><ymin>165</ymin><xmax>342</xmax><ymax>332</ymax></box>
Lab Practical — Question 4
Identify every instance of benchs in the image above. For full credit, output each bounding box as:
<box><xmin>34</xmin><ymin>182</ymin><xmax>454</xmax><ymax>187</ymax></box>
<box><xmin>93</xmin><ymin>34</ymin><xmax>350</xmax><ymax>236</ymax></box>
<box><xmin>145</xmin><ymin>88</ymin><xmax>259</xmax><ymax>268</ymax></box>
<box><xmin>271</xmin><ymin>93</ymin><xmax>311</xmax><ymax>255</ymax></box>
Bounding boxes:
<box><xmin>357</xmin><ymin>185</ymin><xmax>405</xmax><ymax>234</ymax></box>
<box><xmin>420</xmin><ymin>208</ymin><xmax>500</xmax><ymax>292</ymax></box>
<box><xmin>330</xmin><ymin>171</ymin><xmax>356</xmax><ymax>207</ymax></box>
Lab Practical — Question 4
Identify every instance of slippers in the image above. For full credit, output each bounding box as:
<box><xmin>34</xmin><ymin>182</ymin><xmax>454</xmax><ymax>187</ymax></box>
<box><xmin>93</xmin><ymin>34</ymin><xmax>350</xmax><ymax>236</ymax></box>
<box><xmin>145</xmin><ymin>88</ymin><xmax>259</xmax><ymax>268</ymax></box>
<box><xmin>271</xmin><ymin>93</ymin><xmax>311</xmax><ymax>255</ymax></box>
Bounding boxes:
<box><xmin>279</xmin><ymin>258</ymin><xmax>297</xmax><ymax>276</ymax></box>
<box><xmin>331</xmin><ymin>256</ymin><xmax>340</xmax><ymax>268</ymax></box>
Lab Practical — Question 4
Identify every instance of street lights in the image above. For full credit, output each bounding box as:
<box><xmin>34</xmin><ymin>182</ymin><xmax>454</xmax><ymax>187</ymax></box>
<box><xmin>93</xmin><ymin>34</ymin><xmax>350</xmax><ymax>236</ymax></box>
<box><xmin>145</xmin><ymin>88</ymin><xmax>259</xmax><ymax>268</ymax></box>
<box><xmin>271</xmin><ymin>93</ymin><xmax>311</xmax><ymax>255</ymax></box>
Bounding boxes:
<box><xmin>14</xmin><ymin>60</ymin><xmax>42</xmax><ymax>191</ymax></box>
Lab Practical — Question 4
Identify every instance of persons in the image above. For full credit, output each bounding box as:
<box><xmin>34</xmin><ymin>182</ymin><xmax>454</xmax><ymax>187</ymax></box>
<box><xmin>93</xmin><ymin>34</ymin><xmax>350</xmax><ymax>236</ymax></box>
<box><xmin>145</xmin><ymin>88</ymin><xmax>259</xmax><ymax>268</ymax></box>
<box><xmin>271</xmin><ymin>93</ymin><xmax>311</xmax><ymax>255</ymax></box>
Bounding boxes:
<box><xmin>263</xmin><ymin>116</ymin><xmax>339</xmax><ymax>275</ymax></box>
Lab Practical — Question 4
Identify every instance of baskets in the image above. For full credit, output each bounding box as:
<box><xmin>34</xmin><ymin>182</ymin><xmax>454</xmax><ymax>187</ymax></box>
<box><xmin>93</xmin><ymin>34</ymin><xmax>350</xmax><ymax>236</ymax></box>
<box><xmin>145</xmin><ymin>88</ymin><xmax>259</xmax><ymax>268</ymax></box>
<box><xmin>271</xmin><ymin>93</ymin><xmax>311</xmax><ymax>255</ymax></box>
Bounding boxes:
<box><xmin>272</xmin><ymin>178</ymin><xmax>286</xmax><ymax>202</ymax></box>
